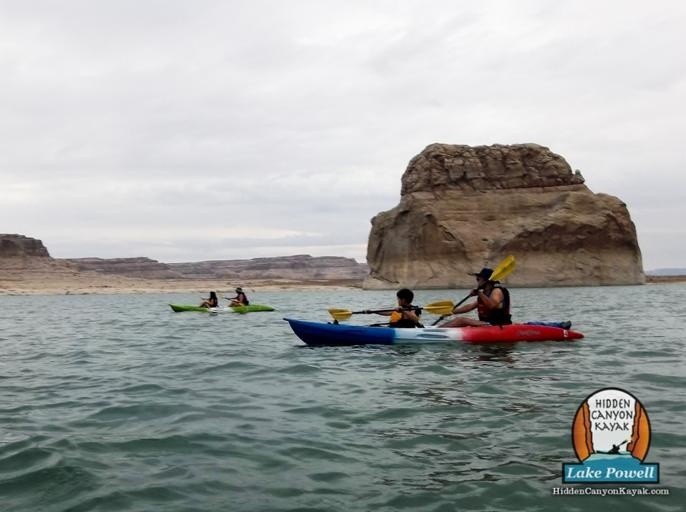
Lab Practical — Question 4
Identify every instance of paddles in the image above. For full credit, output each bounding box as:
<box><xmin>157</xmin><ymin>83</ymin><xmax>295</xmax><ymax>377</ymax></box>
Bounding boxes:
<box><xmin>199</xmin><ymin>295</ymin><xmax>212</xmax><ymax>312</ymax></box>
<box><xmin>327</xmin><ymin>300</ymin><xmax>454</xmax><ymax>322</ymax></box>
<box><xmin>430</xmin><ymin>253</ymin><xmax>522</xmax><ymax>325</ymax></box>
<box><xmin>216</xmin><ymin>292</ymin><xmax>247</xmax><ymax>309</ymax></box>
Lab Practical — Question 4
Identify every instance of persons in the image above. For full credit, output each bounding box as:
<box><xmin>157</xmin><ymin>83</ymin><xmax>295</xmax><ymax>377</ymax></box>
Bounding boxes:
<box><xmin>364</xmin><ymin>288</ymin><xmax>420</xmax><ymax>328</ymax></box>
<box><xmin>436</xmin><ymin>267</ymin><xmax>512</xmax><ymax>327</ymax></box>
<box><xmin>226</xmin><ymin>287</ymin><xmax>249</xmax><ymax>307</ymax></box>
<box><xmin>200</xmin><ymin>292</ymin><xmax>218</xmax><ymax>308</ymax></box>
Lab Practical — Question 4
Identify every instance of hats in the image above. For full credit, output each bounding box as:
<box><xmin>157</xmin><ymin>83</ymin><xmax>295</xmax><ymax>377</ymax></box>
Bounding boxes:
<box><xmin>477</xmin><ymin>268</ymin><xmax>500</xmax><ymax>284</ymax></box>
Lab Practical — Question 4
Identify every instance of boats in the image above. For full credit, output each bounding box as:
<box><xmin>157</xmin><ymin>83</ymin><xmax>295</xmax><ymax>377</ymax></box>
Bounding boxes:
<box><xmin>282</xmin><ymin>318</ymin><xmax>584</xmax><ymax>348</ymax></box>
<box><xmin>169</xmin><ymin>303</ymin><xmax>277</xmax><ymax>315</ymax></box>
